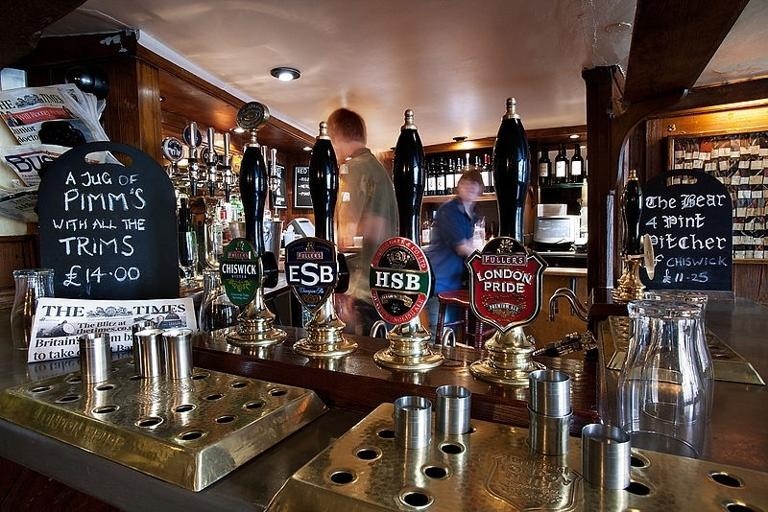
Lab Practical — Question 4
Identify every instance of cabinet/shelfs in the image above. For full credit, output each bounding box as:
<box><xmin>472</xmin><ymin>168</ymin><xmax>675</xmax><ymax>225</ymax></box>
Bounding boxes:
<box><xmin>420</xmin><ymin>126</ymin><xmax>587</xmax><ymax>275</ymax></box>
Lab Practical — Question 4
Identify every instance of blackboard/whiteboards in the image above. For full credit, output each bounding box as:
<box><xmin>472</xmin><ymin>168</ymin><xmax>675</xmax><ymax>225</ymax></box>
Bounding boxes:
<box><xmin>638</xmin><ymin>168</ymin><xmax>732</xmax><ymax>291</ymax></box>
<box><xmin>38</xmin><ymin>140</ymin><xmax>180</xmax><ymax>300</ymax></box>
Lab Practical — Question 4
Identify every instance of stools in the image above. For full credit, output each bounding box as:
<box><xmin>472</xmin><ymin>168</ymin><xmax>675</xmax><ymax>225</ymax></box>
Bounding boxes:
<box><xmin>435</xmin><ymin>289</ymin><xmax>496</xmax><ymax>349</ymax></box>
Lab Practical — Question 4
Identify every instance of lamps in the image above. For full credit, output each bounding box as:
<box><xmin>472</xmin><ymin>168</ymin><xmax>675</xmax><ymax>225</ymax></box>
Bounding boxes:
<box><xmin>271</xmin><ymin>67</ymin><xmax>300</xmax><ymax>82</ymax></box>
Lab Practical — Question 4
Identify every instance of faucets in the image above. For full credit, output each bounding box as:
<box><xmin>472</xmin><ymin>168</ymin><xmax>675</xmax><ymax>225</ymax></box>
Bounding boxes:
<box><xmin>547</xmin><ymin>288</ymin><xmax>588</xmax><ymax>325</ymax></box>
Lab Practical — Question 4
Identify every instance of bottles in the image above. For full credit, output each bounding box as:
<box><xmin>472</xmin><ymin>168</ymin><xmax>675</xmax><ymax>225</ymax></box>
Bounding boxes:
<box><xmin>198</xmin><ymin>269</ymin><xmax>240</xmax><ymax>333</ymax></box>
<box><xmin>555</xmin><ymin>142</ymin><xmax>569</xmax><ymax>184</ymax></box>
<box><xmin>537</xmin><ymin>143</ymin><xmax>552</xmax><ymax>186</ymax></box>
<box><xmin>617</xmin><ymin>293</ymin><xmax>714</xmax><ymax>460</ymax></box>
<box><xmin>423</xmin><ymin>153</ymin><xmax>494</xmax><ymax>195</ymax></box>
<box><xmin>10</xmin><ymin>269</ymin><xmax>56</xmax><ymax>351</ymax></box>
<box><xmin>571</xmin><ymin>144</ymin><xmax>584</xmax><ymax>184</ymax></box>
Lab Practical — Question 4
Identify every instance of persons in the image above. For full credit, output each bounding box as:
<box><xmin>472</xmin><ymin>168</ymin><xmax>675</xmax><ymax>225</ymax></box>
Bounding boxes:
<box><xmin>324</xmin><ymin>105</ymin><xmax>400</xmax><ymax>336</ymax></box>
<box><xmin>424</xmin><ymin>168</ymin><xmax>485</xmax><ymax>345</ymax></box>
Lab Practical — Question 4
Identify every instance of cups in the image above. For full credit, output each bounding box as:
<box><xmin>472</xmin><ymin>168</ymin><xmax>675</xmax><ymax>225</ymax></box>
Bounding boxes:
<box><xmin>436</xmin><ymin>385</ymin><xmax>472</xmax><ymax>435</ymax></box>
<box><xmin>78</xmin><ymin>332</ymin><xmax>112</xmax><ymax>386</ymax></box>
<box><xmin>394</xmin><ymin>396</ymin><xmax>432</xmax><ymax>450</ymax></box>
<box><xmin>132</xmin><ymin>323</ymin><xmax>193</xmax><ymax>380</ymax></box>
<box><xmin>582</xmin><ymin>424</ymin><xmax>631</xmax><ymax>491</ymax></box>
<box><xmin>527</xmin><ymin>370</ymin><xmax>573</xmax><ymax>456</ymax></box>
